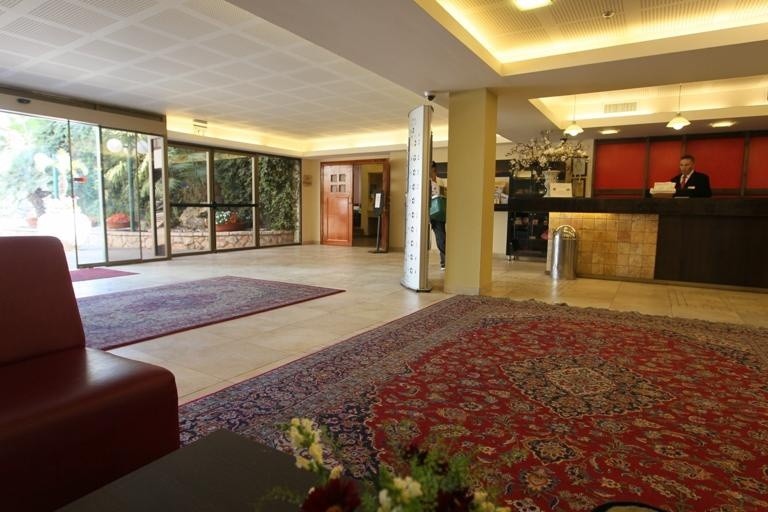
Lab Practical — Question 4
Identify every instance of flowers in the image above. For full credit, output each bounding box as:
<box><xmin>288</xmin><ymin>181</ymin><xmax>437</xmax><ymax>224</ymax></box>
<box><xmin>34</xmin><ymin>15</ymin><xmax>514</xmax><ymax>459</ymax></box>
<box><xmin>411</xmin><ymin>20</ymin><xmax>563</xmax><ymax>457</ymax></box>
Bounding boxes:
<box><xmin>262</xmin><ymin>416</ymin><xmax>513</xmax><ymax>512</ymax></box>
<box><xmin>504</xmin><ymin>129</ymin><xmax>590</xmax><ymax>178</ymax></box>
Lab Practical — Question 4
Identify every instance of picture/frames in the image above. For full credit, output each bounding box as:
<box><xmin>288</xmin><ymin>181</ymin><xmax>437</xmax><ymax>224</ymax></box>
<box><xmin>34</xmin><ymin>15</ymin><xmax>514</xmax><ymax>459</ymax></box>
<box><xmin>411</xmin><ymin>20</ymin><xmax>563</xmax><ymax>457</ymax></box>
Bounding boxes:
<box><xmin>570</xmin><ymin>178</ymin><xmax>586</xmax><ymax>198</ymax></box>
<box><xmin>571</xmin><ymin>156</ymin><xmax>588</xmax><ymax>177</ymax></box>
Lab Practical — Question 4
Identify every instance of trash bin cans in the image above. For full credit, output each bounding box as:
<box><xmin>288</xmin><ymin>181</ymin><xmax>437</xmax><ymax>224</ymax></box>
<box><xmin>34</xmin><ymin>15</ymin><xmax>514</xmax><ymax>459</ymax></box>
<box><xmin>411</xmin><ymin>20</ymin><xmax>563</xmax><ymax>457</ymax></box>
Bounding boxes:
<box><xmin>551</xmin><ymin>225</ymin><xmax>577</xmax><ymax>280</ymax></box>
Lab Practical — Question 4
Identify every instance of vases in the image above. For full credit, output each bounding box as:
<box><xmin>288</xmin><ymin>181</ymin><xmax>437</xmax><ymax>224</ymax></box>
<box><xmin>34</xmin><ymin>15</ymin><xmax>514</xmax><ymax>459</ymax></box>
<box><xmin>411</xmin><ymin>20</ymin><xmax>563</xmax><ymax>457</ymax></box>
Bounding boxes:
<box><xmin>541</xmin><ymin>170</ymin><xmax>560</xmax><ymax>197</ymax></box>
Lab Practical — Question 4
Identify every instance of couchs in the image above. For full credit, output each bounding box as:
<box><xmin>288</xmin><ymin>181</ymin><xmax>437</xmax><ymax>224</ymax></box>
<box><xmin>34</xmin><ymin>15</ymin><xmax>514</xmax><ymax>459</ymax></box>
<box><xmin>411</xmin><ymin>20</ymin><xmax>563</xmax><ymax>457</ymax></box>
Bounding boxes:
<box><xmin>0</xmin><ymin>237</ymin><xmax>180</xmax><ymax>512</ymax></box>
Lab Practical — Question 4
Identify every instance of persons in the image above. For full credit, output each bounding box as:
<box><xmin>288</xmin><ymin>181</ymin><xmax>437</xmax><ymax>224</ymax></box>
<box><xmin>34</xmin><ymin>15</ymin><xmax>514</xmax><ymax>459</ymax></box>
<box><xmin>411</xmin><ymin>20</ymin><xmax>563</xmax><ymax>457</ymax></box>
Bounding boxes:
<box><xmin>429</xmin><ymin>161</ymin><xmax>445</xmax><ymax>271</ymax></box>
<box><xmin>671</xmin><ymin>156</ymin><xmax>712</xmax><ymax>198</ymax></box>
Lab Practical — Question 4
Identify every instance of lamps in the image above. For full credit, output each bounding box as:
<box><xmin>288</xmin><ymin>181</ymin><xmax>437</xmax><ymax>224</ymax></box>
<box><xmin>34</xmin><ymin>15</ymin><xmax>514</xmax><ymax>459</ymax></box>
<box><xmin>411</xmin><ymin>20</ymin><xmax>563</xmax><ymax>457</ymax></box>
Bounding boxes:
<box><xmin>666</xmin><ymin>83</ymin><xmax>691</xmax><ymax>130</ymax></box>
<box><xmin>563</xmin><ymin>94</ymin><xmax>584</xmax><ymax>137</ymax></box>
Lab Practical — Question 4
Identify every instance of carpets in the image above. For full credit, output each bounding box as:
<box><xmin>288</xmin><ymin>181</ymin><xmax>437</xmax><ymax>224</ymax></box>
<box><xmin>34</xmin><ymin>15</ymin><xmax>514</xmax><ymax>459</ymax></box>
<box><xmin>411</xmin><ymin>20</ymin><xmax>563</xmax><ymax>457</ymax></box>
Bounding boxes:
<box><xmin>177</xmin><ymin>293</ymin><xmax>768</xmax><ymax>512</ymax></box>
<box><xmin>66</xmin><ymin>267</ymin><xmax>142</xmax><ymax>283</ymax></box>
<box><xmin>76</xmin><ymin>275</ymin><xmax>348</xmax><ymax>350</ymax></box>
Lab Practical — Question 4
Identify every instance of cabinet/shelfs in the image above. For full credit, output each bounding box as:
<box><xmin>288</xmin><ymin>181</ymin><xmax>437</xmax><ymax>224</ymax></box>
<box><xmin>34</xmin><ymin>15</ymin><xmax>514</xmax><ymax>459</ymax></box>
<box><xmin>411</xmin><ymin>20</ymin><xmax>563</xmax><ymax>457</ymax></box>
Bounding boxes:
<box><xmin>506</xmin><ymin>160</ymin><xmax>565</xmax><ymax>261</ymax></box>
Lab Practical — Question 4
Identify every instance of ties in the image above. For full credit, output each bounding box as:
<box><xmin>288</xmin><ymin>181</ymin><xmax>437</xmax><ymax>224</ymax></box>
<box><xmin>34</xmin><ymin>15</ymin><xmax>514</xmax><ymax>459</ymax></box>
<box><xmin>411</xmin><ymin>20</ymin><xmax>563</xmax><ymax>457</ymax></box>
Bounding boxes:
<box><xmin>681</xmin><ymin>175</ymin><xmax>687</xmax><ymax>188</ymax></box>
